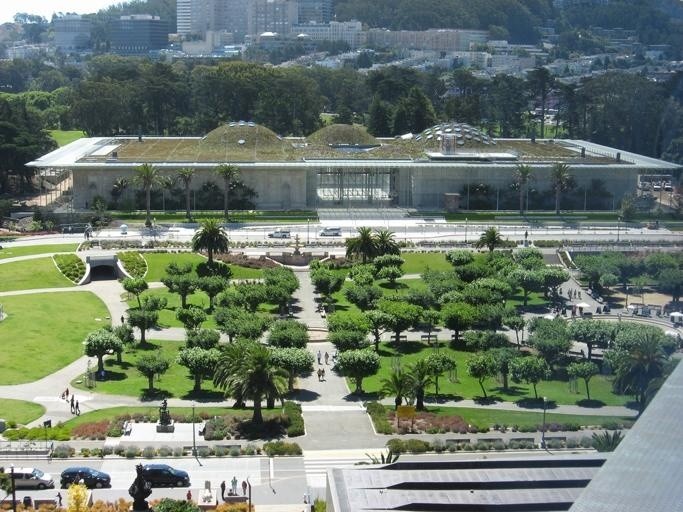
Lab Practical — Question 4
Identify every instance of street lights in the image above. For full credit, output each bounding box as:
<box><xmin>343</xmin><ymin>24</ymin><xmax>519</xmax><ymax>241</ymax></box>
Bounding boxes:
<box><xmin>540</xmin><ymin>397</ymin><xmax>548</xmax><ymax>448</ymax></box>
<box><xmin>190</xmin><ymin>399</ymin><xmax>198</xmax><ymax>456</ymax></box>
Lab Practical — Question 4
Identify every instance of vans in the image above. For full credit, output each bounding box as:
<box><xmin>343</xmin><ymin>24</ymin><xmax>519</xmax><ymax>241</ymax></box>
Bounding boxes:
<box><xmin>320</xmin><ymin>227</ymin><xmax>340</xmax><ymax>237</ymax></box>
<box><xmin>267</xmin><ymin>230</ymin><xmax>290</xmax><ymax>238</ymax></box>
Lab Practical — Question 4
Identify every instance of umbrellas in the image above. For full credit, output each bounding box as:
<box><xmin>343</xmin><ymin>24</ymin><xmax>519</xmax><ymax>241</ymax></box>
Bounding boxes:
<box><xmin>576</xmin><ymin>302</ymin><xmax>591</xmax><ymax>308</ymax></box>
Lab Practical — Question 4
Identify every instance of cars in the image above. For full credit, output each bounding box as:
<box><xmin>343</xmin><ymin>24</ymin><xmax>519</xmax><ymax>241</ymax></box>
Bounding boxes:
<box><xmin>61</xmin><ymin>467</ymin><xmax>110</xmax><ymax>488</ymax></box>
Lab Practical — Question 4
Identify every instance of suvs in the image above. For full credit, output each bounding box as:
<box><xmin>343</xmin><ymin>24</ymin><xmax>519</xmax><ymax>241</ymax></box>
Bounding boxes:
<box><xmin>4</xmin><ymin>467</ymin><xmax>54</xmax><ymax>489</ymax></box>
<box><xmin>143</xmin><ymin>462</ymin><xmax>189</xmax><ymax>489</ymax></box>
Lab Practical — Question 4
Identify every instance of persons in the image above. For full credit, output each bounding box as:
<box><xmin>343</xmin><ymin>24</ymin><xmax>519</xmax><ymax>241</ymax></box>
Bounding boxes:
<box><xmin>186</xmin><ymin>490</ymin><xmax>192</xmax><ymax>500</ymax></box>
<box><xmin>220</xmin><ymin>480</ymin><xmax>225</xmax><ymax>499</ymax></box>
<box><xmin>324</xmin><ymin>351</ymin><xmax>329</xmax><ymax>364</ymax></box>
<box><xmin>241</xmin><ymin>480</ymin><xmax>247</xmax><ymax>495</ymax></box>
<box><xmin>316</xmin><ymin>349</ymin><xmax>321</xmax><ymax>364</ymax></box>
<box><xmin>159</xmin><ymin>399</ymin><xmax>168</xmax><ymax>413</ymax></box>
<box><xmin>120</xmin><ymin>315</ymin><xmax>124</xmax><ymax>324</ymax></box>
<box><xmin>54</xmin><ymin>491</ymin><xmax>62</xmax><ymax>505</ymax></box>
<box><xmin>65</xmin><ymin>388</ymin><xmax>81</xmax><ymax>415</ymax></box>
<box><xmin>230</xmin><ymin>475</ymin><xmax>237</xmax><ymax>495</ymax></box>
<box><xmin>227</xmin><ymin>488</ymin><xmax>234</xmax><ymax>495</ymax></box>
<box><xmin>316</xmin><ymin>368</ymin><xmax>321</xmax><ymax>381</ymax></box>
<box><xmin>321</xmin><ymin>368</ymin><xmax>326</xmax><ymax>380</ymax></box>
<box><xmin>567</xmin><ymin>287</ymin><xmax>581</xmax><ymax>300</ymax></box>
